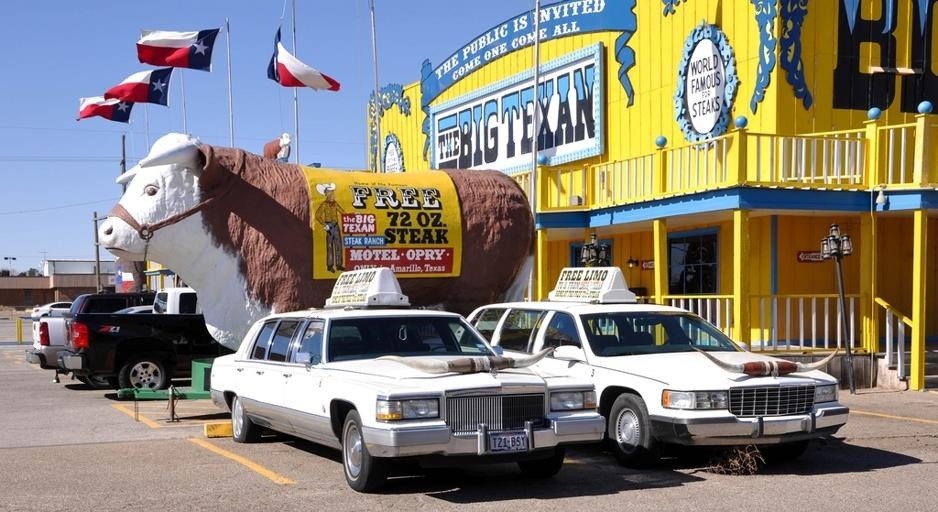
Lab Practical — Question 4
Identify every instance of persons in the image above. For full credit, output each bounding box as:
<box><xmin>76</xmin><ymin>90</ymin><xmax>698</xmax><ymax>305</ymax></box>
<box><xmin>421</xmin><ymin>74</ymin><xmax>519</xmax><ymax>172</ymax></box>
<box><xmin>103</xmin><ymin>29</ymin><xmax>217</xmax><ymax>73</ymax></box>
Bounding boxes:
<box><xmin>314</xmin><ymin>182</ymin><xmax>351</xmax><ymax>273</ymax></box>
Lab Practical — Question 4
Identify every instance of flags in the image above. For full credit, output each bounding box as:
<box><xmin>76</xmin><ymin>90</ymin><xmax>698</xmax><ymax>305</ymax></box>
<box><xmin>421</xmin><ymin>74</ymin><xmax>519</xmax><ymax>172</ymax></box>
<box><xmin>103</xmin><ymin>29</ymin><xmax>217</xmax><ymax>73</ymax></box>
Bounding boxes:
<box><xmin>266</xmin><ymin>24</ymin><xmax>344</xmax><ymax>94</ymax></box>
<box><xmin>103</xmin><ymin>67</ymin><xmax>175</xmax><ymax>108</ymax></box>
<box><xmin>76</xmin><ymin>95</ymin><xmax>136</xmax><ymax>126</ymax></box>
<box><xmin>135</xmin><ymin>25</ymin><xmax>221</xmax><ymax>73</ymax></box>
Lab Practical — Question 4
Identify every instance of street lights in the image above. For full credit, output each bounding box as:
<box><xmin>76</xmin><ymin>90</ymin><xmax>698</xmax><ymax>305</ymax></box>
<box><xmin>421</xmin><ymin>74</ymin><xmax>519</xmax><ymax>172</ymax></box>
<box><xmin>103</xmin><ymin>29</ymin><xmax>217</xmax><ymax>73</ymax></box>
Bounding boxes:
<box><xmin>5</xmin><ymin>256</ymin><xmax>17</xmax><ymax>275</ymax></box>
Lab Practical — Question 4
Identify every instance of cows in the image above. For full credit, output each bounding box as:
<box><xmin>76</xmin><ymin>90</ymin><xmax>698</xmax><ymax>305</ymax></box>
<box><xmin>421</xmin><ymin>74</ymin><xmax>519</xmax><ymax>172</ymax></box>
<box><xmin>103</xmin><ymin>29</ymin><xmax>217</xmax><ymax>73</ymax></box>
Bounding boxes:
<box><xmin>99</xmin><ymin>133</ymin><xmax>537</xmax><ymax>352</ymax></box>
<box><xmin>264</xmin><ymin>133</ymin><xmax>292</xmax><ymax>162</ymax></box>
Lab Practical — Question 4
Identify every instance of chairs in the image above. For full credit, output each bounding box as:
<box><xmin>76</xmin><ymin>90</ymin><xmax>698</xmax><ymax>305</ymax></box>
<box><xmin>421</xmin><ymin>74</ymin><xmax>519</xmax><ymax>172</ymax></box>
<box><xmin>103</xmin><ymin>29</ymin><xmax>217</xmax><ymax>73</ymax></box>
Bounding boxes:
<box><xmin>594</xmin><ymin>332</ymin><xmax>653</xmax><ymax>354</ymax></box>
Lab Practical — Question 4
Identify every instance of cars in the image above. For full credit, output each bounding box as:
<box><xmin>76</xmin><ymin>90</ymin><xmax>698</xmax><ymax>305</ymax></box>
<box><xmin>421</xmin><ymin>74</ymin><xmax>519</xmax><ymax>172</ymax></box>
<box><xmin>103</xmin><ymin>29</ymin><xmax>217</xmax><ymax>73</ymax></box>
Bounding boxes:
<box><xmin>216</xmin><ymin>268</ymin><xmax>606</xmax><ymax>490</ymax></box>
<box><xmin>456</xmin><ymin>268</ymin><xmax>848</xmax><ymax>462</ymax></box>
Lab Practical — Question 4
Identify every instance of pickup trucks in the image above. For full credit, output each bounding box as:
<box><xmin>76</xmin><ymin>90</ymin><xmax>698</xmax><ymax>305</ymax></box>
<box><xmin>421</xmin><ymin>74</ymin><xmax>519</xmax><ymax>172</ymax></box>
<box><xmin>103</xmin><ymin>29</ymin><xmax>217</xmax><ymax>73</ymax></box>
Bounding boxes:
<box><xmin>32</xmin><ymin>282</ymin><xmax>220</xmax><ymax>390</ymax></box>
